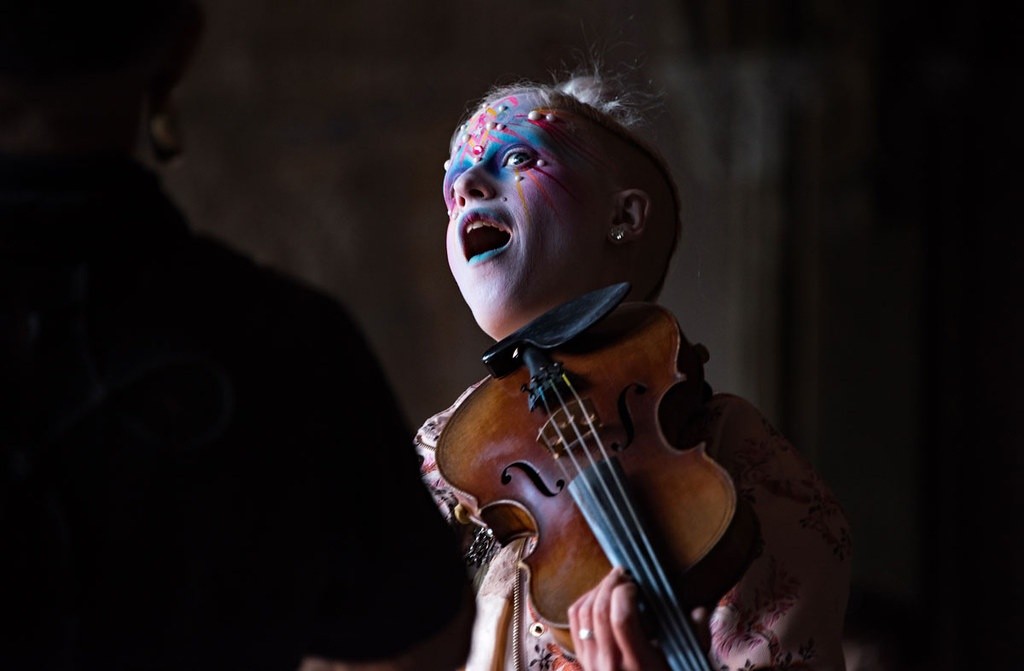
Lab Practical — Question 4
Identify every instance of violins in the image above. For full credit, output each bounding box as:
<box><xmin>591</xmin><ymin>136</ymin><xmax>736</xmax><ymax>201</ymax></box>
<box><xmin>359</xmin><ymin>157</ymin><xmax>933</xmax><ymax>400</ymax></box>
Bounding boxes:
<box><xmin>431</xmin><ymin>303</ymin><xmax>758</xmax><ymax>671</ymax></box>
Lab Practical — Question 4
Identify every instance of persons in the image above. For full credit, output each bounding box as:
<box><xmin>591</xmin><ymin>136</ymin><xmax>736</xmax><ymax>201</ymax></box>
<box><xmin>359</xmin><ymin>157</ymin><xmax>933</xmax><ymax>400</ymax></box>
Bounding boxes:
<box><xmin>411</xmin><ymin>23</ymin><xmax>849</xmax><ymax>671</ymax></box>
<box><xmin>0</xmin><ymin>0</ymin><xmax>475</xmax><ymax>671</ymax></box>
